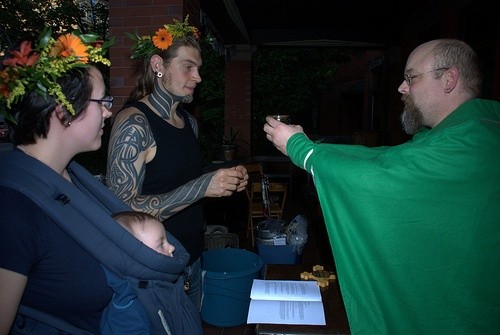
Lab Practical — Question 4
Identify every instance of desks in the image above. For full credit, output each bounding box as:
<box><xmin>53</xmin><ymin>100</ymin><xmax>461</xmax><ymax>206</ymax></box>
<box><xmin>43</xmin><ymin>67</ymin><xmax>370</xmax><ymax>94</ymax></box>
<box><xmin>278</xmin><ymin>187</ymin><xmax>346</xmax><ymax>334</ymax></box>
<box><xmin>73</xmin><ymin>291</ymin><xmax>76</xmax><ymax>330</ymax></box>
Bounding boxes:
<box><xmin>256</xmin><ymin>264</ymin><xmax>350</xmax><ymax>335</ymax></box>
<box><xmin>244</xmin><ymin>156</ymin><xmax>293</xmax><ymax>197</ymax></box>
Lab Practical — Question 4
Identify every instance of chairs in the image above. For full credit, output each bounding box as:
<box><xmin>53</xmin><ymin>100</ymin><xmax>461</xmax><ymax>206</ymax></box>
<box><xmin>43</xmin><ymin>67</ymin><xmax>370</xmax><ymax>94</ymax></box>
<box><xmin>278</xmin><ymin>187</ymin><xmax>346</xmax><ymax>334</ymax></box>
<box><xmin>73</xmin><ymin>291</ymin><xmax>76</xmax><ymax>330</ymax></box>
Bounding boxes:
<box><xmin>246</xmin><ymin>181</ymin><xmax>289</xmax><ymax>248</ymax></box>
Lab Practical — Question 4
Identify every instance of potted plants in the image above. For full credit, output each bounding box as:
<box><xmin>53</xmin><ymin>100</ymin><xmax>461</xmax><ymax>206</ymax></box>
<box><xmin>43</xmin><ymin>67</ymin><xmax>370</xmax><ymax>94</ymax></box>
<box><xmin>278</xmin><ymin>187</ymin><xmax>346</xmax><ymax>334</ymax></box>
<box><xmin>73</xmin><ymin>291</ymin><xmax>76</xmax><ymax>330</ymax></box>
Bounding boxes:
<box><xmin>221</xmin><ymin>126</ymin><xmax>250</xmax><ymax>161</ymax></box>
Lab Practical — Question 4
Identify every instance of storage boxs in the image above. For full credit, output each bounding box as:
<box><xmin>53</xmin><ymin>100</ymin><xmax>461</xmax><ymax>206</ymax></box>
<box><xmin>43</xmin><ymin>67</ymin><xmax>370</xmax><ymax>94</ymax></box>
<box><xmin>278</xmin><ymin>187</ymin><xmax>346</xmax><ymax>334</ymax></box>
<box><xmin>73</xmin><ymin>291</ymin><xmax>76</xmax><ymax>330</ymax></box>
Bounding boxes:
<box><xmin>257</xmin><ymin>244</ymin><xmax>297</xmax><ymax>264</ymax></box>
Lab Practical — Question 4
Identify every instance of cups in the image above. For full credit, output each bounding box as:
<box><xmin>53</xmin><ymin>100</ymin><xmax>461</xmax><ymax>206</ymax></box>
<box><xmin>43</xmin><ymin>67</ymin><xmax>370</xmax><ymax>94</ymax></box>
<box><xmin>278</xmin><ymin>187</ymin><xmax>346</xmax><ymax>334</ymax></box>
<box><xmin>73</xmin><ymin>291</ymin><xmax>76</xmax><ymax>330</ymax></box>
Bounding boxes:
<box><xmin>270</xmin><ymin>114</ymin><xmax>291</xmax><ymax>128</ymax></box>
<box><xmin>206</xmin><ymin>233</ymin><xmax>239</xmax><ymax>249</ymax></box>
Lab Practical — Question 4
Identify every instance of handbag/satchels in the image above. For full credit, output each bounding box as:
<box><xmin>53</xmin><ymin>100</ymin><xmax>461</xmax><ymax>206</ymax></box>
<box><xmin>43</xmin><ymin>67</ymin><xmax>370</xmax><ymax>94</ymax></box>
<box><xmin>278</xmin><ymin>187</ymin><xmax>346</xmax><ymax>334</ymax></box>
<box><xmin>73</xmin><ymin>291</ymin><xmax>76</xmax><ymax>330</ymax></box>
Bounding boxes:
<box><xmin>204</xmin><ymin>227</ymin><xmax>240</xmax><ymax>249</ymax></box>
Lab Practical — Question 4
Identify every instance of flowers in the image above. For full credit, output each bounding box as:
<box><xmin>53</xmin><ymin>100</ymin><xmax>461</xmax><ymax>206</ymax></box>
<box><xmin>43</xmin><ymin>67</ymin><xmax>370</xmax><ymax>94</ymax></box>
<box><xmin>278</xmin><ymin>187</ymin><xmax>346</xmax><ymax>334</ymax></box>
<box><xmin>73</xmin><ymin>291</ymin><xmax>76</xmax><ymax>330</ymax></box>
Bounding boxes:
<box><xmin>122</xmin><ymin>12</ymin><xmax>202</xmax><ymax>60</ymax></box>
<box><xmin>0</xmin><ymin>25</ymin><xmax>111</xmax><ymax>125</ymax></box>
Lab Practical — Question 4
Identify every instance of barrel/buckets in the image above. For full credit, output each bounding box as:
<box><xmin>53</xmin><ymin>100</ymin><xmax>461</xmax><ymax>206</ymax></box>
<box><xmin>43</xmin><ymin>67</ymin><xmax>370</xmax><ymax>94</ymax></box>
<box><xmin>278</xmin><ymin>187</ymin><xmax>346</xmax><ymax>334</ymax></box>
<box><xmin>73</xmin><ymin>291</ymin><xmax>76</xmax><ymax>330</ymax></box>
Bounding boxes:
<box><xmin>198</xmin><ymin>247</ymin><xmax>267</xmax><ymax>327</ymax></box>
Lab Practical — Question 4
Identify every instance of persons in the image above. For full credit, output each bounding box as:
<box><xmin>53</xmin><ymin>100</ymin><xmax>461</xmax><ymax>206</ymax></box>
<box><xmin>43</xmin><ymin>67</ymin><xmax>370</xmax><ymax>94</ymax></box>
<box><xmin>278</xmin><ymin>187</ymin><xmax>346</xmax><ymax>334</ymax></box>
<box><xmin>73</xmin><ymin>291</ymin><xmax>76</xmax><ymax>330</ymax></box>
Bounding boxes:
<box><xmin>106</xmin><ymin>22</ymin><xmax>249</xmax><ymax>313</ymax></box>
<box><xmin>264</xmin><ymin>38</ymin><xmax>500</xmax><ymax>335</ymax></box>
<box><xmin>0</xmin><ymin>32</ymin><xmax>205</xmax><ymax>335</ymax></box>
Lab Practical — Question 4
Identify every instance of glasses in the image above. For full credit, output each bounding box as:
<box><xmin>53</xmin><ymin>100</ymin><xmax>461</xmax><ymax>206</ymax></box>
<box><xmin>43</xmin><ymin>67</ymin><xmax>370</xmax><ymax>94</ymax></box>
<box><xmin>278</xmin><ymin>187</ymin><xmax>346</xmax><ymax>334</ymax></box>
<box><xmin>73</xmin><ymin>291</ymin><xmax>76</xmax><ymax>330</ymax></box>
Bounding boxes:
<box><xmin>404</xmin><ymin>68</ymin><xmax>450</xmax><ymax>86</ymax></box>
<box><xmin>86</xmin><ymin>95</ymin><xmax>115</xmax><ymax>109</ymax></box>
